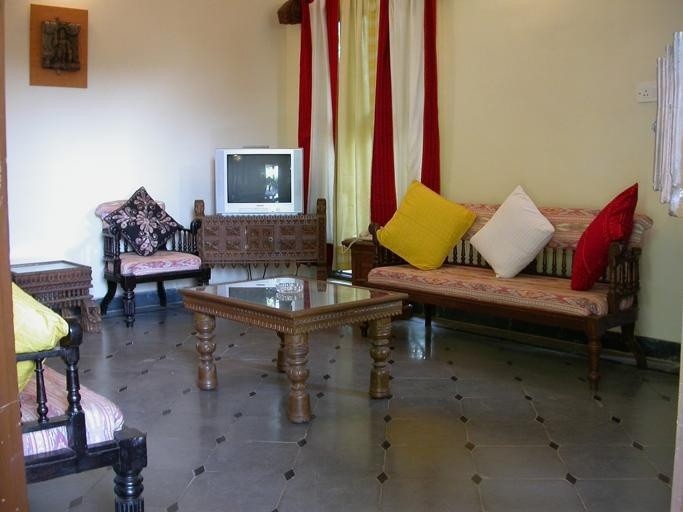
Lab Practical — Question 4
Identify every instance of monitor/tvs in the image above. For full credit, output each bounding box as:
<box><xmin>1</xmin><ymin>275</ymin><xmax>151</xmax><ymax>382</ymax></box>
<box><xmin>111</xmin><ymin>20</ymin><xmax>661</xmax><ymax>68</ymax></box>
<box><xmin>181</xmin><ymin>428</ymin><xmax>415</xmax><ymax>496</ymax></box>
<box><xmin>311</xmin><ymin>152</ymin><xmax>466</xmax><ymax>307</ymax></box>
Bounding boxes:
<box><xmin>215</xmin><ymin>148</ymin><xmax>304</xmax><ymax>216</ymax></box>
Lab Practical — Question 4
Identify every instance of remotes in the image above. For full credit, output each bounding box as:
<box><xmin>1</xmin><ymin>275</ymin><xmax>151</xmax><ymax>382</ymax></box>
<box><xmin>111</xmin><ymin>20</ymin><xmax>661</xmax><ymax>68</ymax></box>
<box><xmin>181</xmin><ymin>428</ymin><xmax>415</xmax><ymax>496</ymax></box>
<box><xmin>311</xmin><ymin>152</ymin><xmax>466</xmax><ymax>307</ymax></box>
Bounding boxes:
<box><xmin>243</xmin><ymin>144</ymin><xmax>269</xmax><ymax>148</ymax></box>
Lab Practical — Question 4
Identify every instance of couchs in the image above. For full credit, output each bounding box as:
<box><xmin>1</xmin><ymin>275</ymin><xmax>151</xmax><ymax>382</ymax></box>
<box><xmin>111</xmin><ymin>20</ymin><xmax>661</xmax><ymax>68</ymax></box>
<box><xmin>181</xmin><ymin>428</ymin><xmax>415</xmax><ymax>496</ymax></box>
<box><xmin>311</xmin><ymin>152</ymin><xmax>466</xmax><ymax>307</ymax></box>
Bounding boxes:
<box><xmin>369</xmin><ymin>200</ymin><xmax>655</xmax><ymax>391</ymax></box>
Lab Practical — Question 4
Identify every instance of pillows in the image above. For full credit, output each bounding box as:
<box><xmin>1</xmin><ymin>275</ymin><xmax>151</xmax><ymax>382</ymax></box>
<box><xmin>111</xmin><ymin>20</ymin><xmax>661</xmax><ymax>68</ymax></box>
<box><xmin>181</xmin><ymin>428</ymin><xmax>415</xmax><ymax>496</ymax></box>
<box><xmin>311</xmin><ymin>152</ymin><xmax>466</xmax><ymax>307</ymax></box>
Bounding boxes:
<box><xmin>101</xmin><ymin>187</ymin><xmax>184</xmax><ymax>256</ymax></box>
<box><xmin>8</xmin><ymin>281</ymin><xmax>73</xmax><ymax>395</ymax></box>
<box><xmin>374</xmin><ymin>179</ymin><xmax>642</xmax><ymax>292</ymax></box>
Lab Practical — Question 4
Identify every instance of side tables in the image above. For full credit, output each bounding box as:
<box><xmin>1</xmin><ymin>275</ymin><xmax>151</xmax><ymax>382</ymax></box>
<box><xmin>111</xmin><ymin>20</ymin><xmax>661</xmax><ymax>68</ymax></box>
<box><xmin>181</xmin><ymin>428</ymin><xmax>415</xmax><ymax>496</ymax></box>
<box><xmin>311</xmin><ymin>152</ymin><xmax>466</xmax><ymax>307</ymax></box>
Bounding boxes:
<box><xmin>10</xmin><ymin>259</ymin><xmax>105</xmax><ymax>334</ymax></box>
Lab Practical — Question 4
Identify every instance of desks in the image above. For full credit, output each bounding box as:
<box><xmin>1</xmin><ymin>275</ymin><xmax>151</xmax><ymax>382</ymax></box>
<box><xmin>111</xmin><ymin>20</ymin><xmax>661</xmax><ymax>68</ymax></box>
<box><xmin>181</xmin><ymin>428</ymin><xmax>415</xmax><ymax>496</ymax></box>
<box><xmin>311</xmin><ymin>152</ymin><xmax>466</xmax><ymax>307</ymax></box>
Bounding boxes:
<box><xmin>191</xmin><ymin>197</ymin><xmax>329</xmax><ymax>285</ymax></box>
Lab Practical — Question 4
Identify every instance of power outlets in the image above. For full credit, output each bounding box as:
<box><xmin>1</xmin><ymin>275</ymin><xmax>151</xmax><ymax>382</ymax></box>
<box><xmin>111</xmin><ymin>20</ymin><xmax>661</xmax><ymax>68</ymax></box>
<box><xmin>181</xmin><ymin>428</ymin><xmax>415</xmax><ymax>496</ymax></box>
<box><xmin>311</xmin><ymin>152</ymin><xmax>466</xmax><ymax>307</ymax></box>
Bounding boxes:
<box><xmin>636</xmin><ymin>83</ymin><xmax>657</xmax><ymax>103</ymax></box>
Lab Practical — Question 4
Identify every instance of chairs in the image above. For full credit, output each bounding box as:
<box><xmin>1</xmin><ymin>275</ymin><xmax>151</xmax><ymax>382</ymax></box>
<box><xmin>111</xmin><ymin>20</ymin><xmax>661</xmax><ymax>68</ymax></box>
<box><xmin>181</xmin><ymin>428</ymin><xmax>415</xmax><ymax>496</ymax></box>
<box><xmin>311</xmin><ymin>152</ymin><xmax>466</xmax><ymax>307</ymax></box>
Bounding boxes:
<box><xmin>96</xmin><ymin>199</ymin><xmax>211</xmax><ymax>328</ymax></box>
<box><xmin>9</xmin><ymin>276</ymin><xmax>146</xmax><ymax>511</ymax></box>
